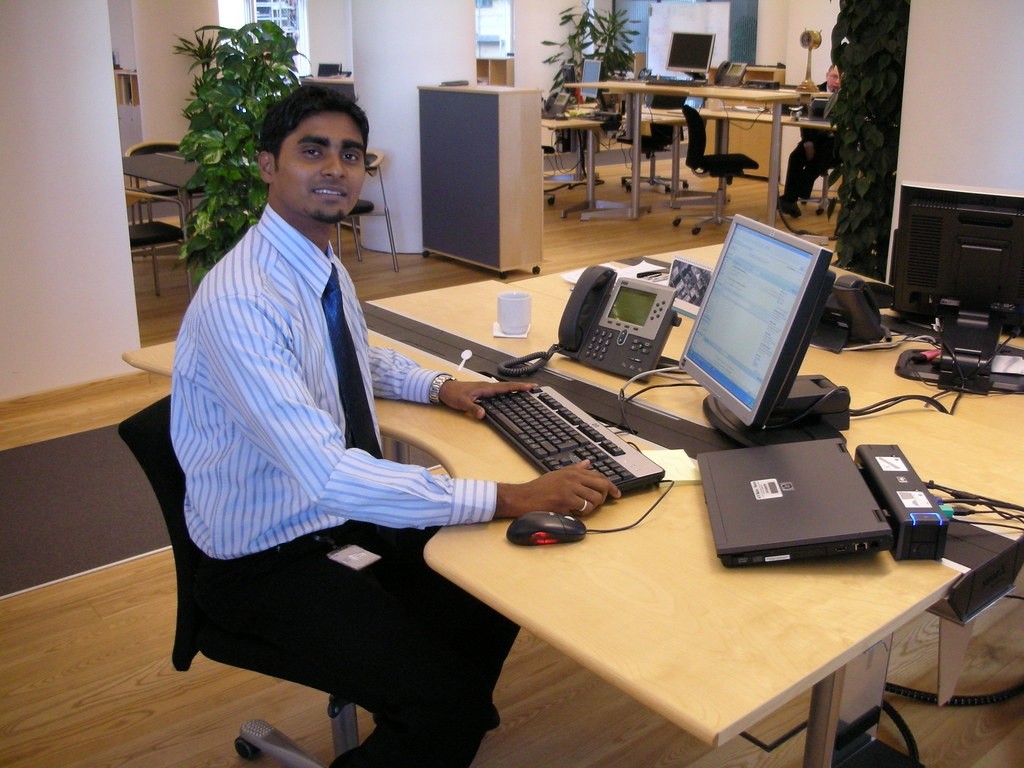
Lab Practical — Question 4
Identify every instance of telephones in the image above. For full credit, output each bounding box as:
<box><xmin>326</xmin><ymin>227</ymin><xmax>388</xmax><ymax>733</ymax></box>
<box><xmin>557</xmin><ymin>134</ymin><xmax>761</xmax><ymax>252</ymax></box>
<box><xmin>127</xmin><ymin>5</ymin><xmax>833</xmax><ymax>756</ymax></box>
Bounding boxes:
<box><xmin>559</xmin><ymin>265</ymin><xmax>684</xmax><ymax>383</ymax></box>
<box><xmin>541</xmin><ymin>91</ymin><xmax>571</xmax><ymax>121</ymax></box>
<box><xmin>713</xmin><ymin>61</ymin><xmax>748</xmax><ymax>87</ymax></box>
<box><xmin>638</xmin><ymin>66</ymin><xmax>653</xmax><ymax>80</ymax></box>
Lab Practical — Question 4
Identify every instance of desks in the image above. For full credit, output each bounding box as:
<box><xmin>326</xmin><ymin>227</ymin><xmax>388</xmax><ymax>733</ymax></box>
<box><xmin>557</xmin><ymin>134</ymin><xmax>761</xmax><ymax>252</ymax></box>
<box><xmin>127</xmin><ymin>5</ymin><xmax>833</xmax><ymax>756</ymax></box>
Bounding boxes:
<box><xmin>363</xmin><ymin>240</ymin><xmax>1024</xmax><ymax>625</ymax></box>
<box><xmin>120</xmin><ymin>329</ymin><xmax>1024</xmax><ymax>768</ymax></box>
<box><xmin>541</xmin><ymin>79</ymin><xmax>845</xmax><ymax>244</ymax></box>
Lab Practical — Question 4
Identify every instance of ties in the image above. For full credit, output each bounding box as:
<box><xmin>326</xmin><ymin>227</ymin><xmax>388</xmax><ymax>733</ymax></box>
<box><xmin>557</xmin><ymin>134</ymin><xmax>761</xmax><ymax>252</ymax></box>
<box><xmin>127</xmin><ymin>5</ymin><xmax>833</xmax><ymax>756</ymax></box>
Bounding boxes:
<box><xmin>322</xmin><ymin>263</ymin><xmax>382</xmax><ymax>460</ymax></box>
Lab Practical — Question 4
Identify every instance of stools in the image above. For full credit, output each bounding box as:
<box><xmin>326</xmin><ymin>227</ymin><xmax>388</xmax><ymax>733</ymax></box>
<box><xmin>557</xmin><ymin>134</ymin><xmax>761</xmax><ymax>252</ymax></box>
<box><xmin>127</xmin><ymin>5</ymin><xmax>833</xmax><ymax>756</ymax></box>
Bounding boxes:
<box><xmin>798</xmin><ymin>166</ymin><xmax>854</xmax><ymax>215</ymax></box>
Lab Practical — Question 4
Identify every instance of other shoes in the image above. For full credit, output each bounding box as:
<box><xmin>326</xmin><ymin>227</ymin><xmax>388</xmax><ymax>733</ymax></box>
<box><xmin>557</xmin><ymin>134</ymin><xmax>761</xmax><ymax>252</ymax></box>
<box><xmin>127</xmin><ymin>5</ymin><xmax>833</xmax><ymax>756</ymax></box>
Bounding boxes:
<box><xmin>777</xmin><ymin>193</ymin><xmax>803</xmax><ymax>218</ymax></box>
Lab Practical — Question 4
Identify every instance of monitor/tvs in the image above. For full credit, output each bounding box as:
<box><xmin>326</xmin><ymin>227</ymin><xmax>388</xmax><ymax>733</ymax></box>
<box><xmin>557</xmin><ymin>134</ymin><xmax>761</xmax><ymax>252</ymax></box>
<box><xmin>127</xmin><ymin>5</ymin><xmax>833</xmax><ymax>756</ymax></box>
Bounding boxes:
<box><xmin>892</xmin><ymin>183</ymin><xmax>1024</xmax><ymax>395</ymax></box>
<box><xmin>563</xmin><ymin>58</ymin><xmax>614</xmax><ymax>121</ymax></box>
<box><xmin>318</xmin><ymin>64</ymin><xmax>352</xmax><ymax>78</ymax></box>
<box><xmin>680</xmin><ymin>214</ymin><xmax>851</xmax><ymax>448</ymax></box>
<box><xmin>665</xmin><ymin>31</ymin><xmax>716</xmax><ymax>83</ymax></box>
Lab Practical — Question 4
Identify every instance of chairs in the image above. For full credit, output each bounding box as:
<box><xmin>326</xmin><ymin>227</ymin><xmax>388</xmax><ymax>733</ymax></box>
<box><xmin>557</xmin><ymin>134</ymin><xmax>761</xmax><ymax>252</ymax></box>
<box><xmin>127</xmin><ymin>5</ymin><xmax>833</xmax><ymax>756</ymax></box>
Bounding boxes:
<box><xmin>672</xmin><ymin>104</ymin><xmax>758</xmax><ymax>234</ymax></box>
<box><xmin>123</xmin><ymin>186</ymin><xmax>193</xmax><ymax>302</ymax></box>
<box><xmin>125</xmin><ymin>141</ymin><xmax>192</xmax><ymax>259</ymax></box>
<box><xmin>616</xmin><ymin>95</ymin><xmax>689</xmax><ymax>194</ymax></box>
<box><xmin>330</xmin><ymin>151</ymin><xmax>401</xmax><ymax>274</ymax></box>
<box><xmin>117</xmin><ymin>394</ymin><xmax>371</xmax><ymax>768</ymax></box>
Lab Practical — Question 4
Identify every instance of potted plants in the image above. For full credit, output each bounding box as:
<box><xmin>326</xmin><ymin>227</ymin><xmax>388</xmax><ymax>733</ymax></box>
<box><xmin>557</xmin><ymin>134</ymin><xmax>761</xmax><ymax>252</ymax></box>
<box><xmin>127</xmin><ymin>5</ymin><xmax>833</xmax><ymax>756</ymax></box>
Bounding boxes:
<box><xmin>541</xmin><ymin>1</ymin><xmax>640</xmax><ymax>116</ymax></box>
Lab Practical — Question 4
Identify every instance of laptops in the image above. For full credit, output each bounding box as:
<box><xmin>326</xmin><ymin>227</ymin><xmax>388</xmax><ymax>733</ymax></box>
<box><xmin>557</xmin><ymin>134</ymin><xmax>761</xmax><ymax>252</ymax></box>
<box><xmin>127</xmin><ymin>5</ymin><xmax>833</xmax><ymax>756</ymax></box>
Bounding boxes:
<box><xmin>696</xmin><ymin>437</ymin><xmax>894</xmax><ymax>569</ymax></box>
<box><xmin>667</xmin><ymin>95</ymin><xmax>706</xmax><ymax>114</ymax></box>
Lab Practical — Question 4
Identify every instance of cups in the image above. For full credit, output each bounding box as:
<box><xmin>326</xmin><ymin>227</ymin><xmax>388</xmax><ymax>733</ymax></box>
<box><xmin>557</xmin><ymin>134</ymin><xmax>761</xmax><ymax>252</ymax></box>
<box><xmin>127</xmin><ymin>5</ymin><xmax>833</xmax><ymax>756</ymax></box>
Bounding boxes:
<box><xmin>497</xmin><ymin>291</ymin><xmax>532</xmax><ymax>335</ymax></box>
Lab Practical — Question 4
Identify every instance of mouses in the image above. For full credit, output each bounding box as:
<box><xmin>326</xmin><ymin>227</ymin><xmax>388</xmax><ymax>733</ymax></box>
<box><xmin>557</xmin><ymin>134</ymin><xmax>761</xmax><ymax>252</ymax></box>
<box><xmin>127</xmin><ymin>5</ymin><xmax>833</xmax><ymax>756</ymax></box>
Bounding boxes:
<box><xmin>506</xmin><ymin>509</ymin><xmax>586</xmax><ymax>546</ymax></box>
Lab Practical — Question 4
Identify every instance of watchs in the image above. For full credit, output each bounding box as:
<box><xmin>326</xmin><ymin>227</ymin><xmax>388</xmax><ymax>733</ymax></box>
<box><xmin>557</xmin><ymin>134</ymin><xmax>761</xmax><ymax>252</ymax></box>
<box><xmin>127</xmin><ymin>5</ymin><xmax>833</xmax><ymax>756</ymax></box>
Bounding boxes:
<box><xmin>430</xmin><ymin>374</ymin><xmax>456</xmax><ymax>405</ymax></box>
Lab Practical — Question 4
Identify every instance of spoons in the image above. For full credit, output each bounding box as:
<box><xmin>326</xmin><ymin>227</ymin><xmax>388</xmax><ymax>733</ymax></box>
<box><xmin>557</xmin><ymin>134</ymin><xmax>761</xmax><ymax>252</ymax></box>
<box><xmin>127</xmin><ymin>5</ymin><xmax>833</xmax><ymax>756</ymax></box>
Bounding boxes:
<box><xmin>458</xmin><ymin>349</ymin><xmax>473</xmax><ymax>371</ymax></box>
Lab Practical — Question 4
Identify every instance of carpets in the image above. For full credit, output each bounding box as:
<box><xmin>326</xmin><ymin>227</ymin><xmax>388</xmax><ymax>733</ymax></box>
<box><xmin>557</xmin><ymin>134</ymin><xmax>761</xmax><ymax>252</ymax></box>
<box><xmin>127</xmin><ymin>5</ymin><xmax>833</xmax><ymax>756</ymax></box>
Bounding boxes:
<box><xmin>0</xmin><ymin>425</ymin><xmax>173</xmax><ymax>598</ymax></box>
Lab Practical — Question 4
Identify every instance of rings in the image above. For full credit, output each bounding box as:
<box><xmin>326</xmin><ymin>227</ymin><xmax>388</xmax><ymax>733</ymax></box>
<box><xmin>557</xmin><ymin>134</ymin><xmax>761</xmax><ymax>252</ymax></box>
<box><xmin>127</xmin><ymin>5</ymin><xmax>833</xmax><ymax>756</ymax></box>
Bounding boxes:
<box><xmin>579</xmin><ymin>499</ymin><xmax>587</xmax><ymax>511</ymax></box>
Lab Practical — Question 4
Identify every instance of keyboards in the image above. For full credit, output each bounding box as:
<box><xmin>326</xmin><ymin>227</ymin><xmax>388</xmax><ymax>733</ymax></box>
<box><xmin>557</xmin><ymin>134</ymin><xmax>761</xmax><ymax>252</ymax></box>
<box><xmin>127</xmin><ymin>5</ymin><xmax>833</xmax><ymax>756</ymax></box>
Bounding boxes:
<box><xmin>646</xmin><ymin>79</ymin><xmax>706</xmax><ymax>88</ymax></box>
<box><xmin>473</xmin><ymin>385</ymin><xmax>665</xmax><ymax>493</ymax></box>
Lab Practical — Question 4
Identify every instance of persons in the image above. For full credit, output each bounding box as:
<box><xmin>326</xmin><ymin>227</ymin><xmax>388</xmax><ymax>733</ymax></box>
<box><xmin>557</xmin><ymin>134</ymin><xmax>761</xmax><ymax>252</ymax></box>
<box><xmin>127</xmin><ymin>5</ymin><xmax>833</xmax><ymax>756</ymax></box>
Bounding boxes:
<box><xmin>778</xmin><ymin>65</ymin><xmax>841</xmax><ymax>216</ymax></box>
<box><xmin>169</xmin><ymin>89</ymin><xmax>621</xmax><ymax>768</ymax></box>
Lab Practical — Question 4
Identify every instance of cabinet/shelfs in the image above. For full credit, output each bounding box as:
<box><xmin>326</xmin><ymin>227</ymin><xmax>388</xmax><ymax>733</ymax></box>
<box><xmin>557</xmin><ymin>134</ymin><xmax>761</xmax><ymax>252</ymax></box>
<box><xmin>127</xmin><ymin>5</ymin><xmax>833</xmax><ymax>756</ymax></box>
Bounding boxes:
<box><xmin>417</xmin><ymin>83</ymin><xmax>544</xmax><ymax>278</ymax></box>
<box><xmin>115</xmin><ymin>71</ymin><xmax>144</xmax><ymax>156</ymax></box>
